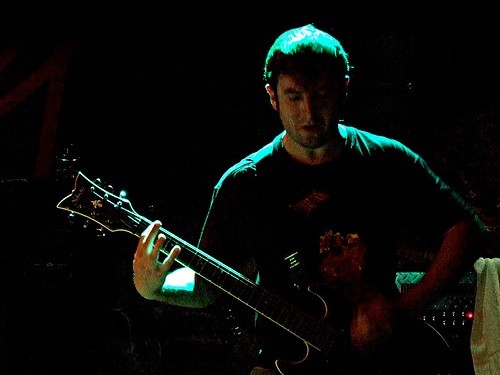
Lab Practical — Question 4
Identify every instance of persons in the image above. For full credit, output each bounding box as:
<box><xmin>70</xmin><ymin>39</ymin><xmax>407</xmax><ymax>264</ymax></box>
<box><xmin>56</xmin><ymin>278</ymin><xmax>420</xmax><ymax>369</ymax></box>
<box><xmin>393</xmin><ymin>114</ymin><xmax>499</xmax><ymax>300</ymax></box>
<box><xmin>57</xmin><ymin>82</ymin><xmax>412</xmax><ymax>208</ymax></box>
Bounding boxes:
<box><xmin>132</xmin><ymin>25</ymin><xmax>490</xmax><ymax>374</ymax></box>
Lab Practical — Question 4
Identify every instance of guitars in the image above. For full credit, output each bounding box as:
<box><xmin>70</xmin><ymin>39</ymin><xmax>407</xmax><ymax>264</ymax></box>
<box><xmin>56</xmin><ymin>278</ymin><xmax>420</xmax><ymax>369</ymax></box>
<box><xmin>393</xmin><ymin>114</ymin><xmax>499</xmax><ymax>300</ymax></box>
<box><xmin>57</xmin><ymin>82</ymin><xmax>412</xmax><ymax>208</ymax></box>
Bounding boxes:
<box><xmin>54</xmin><ymin>169</ymin><xmax>466</xmax><ymax>374</ymax></box>
